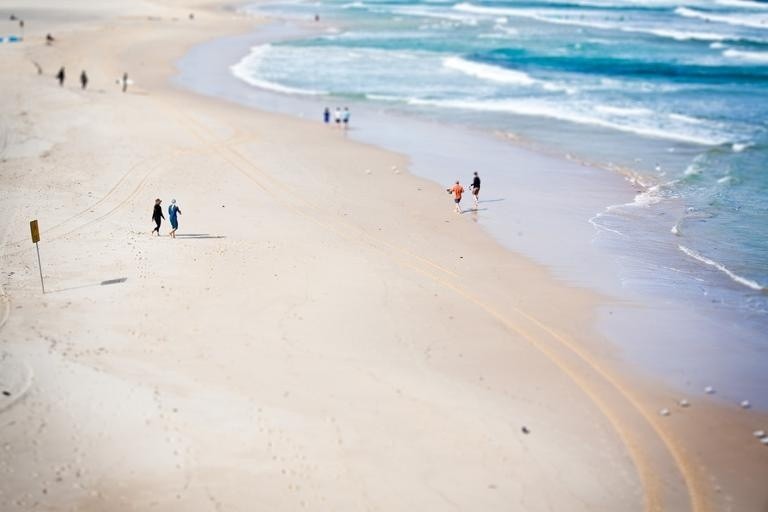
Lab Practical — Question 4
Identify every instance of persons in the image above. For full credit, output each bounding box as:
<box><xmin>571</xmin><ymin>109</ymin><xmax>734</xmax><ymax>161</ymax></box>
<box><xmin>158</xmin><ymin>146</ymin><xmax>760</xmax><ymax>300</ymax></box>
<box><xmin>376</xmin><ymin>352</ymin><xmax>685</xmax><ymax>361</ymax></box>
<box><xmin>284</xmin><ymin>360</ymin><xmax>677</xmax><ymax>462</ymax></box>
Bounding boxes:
<box><xmin>80</xmin><ymin>70</ymin><xmax>87</xmax><ymax>89</ymax></box>
<box><xmin>168</xmin><ymin>200</ymin><xmax>182</xmax><ymax>239</ymax></box>
<box><xmin>151</xmin><ymin>198</ymin><xmax>165</xmax><ymax>236</ymax></box>
<box><xmin>335</xmin><ymin>106</ymin><xmax>341</xmax><ymax>128</ymax></box>
<box><xmin>322</xmin><ymin>107</ymin><xmax>330</xmax><ymax>124</ymax></box>
<box><xmin>57</xmin><ymin>66</ymin><xmax>65</xmax><ymax>84</ymax></box>
<box><xmin>449</xmin><ymin>180</ymin><xmax>464</xmax><ymax>214</ymax></box>
<box><xmin>343</xmin><ymin>107</ymin><xmax>350</xmax><ymax>129</ymax></box>
<box><xmin>121</xmin><ymin>72</ymin><xmax>128</xmax><ymax>93</ymax></box>
<box><xmin>471</xmin><ymin>171</ymin><xmax>480</xmax><ymax>211</ymax></box>
